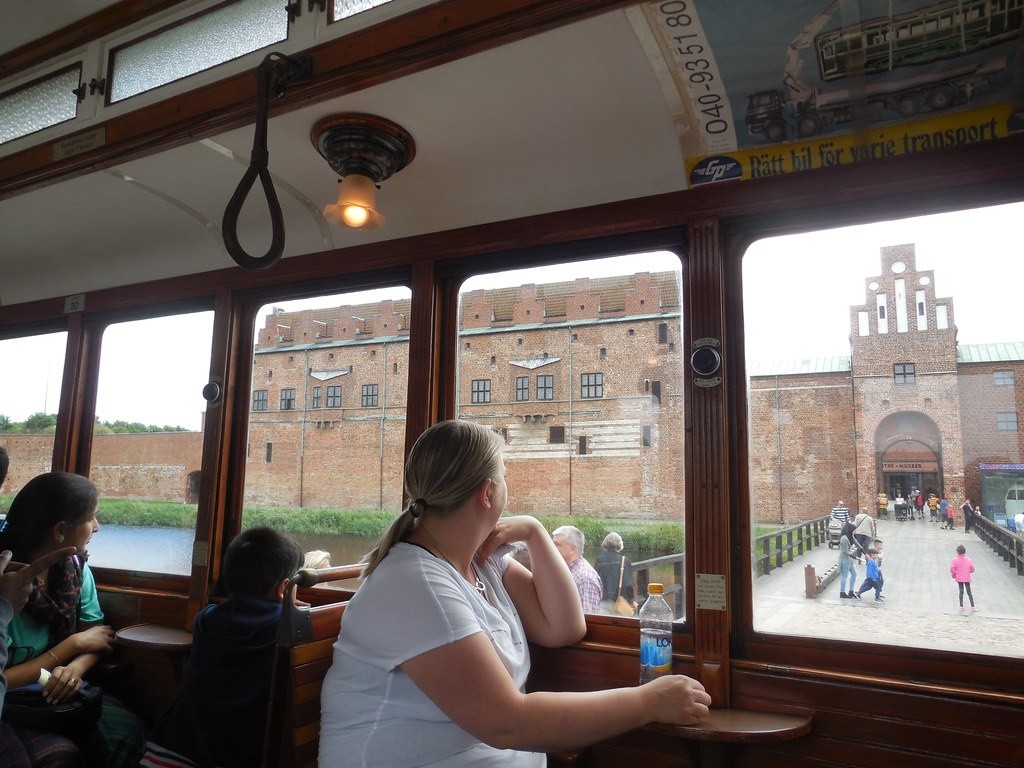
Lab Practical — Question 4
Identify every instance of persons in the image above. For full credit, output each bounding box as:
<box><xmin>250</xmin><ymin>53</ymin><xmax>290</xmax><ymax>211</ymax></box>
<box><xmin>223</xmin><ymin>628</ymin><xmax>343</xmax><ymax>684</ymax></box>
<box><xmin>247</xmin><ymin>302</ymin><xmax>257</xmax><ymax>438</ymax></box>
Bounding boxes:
<box><xmin>317</xmin><ymin>414</ymin><xmax>712</xmax><ymax>768</ymax></box>
<box><xmin>927</xmin><ymin>493</ymin><xmax>955</xmax><ymax>530</ymax></box>
<box><xmin>891</xmin><ymin>483</ymin><xmax>925</xmax><ymax>521</ymax></box>
<box><xmin>831</xmin><ymin>492</ymin><xmax>888</xmax><ymax>604</ymax></box>
<box><xmin>1010</xmin><ymin>512</ymin><xmax>1024</xmax><ymax>537</ymax></box>
<box><xmin>962</xmin><ymin>499</ymin><xmax>982</xmax><ymax>534</ymax></box>
<box><xmin>160</xmin><ymin>527</ymin><xmax>311</xmax><ymax>768</ymax></box>
<box><xmin>0</xmin><ymin>446</ymin><xmax>148</xmax><ymax>768</ymax></box>
<box><xmin>950</xmin><ymin>545</ymin><xmax>978</xmax><ymax>612</ymax></box>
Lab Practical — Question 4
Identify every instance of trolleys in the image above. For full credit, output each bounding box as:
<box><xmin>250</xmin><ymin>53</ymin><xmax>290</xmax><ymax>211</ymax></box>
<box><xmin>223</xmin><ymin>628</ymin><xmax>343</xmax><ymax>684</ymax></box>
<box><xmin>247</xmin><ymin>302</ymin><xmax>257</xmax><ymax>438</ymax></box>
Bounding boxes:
<box><xmin>895</xmin><ymin>505</ymin><xmax>908</xmax><ymax>520</ymax></box>
<box><xmin>994</xmin><ymin>512</ymin><xmax>1007</xmax><ymax>528</ymax></box>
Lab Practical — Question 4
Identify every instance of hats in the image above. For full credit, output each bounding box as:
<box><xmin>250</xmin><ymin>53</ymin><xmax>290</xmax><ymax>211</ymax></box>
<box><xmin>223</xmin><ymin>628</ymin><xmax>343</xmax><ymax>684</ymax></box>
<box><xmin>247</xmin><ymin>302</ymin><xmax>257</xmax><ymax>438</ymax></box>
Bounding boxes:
<box><xmin>879</xmin><ymin>492</ymin><xmax>886</xmax><ymax>497</ymax></box>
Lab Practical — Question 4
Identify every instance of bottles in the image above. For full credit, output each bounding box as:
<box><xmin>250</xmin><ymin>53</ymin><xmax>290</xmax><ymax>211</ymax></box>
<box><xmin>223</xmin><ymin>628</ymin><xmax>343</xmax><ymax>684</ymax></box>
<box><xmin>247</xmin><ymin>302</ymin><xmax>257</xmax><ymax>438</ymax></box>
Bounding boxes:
<box><xmin>638</xmin><ymin>582</ymin><xmax>674</xmax><ymax>689</ymax></box>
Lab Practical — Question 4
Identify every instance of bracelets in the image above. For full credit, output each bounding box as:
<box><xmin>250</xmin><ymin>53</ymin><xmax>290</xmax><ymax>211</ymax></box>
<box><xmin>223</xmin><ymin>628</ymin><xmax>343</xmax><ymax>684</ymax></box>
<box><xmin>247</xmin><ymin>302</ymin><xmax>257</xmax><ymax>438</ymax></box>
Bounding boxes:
<box><xmin>45</xmin><ymin>650</ymin><xmax>61</xmax><ymax>667</ymax></box>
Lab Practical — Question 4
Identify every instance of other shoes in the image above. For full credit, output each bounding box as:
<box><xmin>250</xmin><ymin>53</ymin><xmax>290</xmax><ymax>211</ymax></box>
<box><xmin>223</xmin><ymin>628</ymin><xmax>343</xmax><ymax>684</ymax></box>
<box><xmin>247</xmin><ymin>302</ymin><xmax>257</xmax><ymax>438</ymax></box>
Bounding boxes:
<box><xmin>854</xmin><ymin>591</ymin><xmax>861</xmax><ymax>600</ymax></box>
<box><xmin>879</xmin><ymin>594</ymin><xmax>886</xmax><ymax>598</ymax></box>
<box><xmin>875</xmin><ymin>598</ymin><xmax>884</xmax><ymax>604</ymax></box>
<box><xmin>960</xmin><ymin>606</ymin><xmax>964</xmax><ymax>612</ymax></box>
<box><xmin>972</xmin><ymin>605</ymin><xmax>977</xmax><ymax>612</ymax></box>
<box><xmin>841</xmin><ymin>592</ymin><xmax>850</xmax><ymax>598</ymax></box>
<box><xmin>849</xmin><ymin>591</ymin><xmax>859</xmax><ymax>599</ymax></box>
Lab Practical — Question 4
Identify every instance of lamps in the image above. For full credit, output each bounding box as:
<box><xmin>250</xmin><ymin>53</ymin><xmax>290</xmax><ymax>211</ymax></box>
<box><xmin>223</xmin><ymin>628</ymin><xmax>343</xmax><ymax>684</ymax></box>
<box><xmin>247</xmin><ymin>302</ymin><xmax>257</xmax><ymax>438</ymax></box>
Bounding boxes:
<box><xmin>309</xmin><ymin>113</ymin><xmax>415</xmax><ymax>235</ymax></box>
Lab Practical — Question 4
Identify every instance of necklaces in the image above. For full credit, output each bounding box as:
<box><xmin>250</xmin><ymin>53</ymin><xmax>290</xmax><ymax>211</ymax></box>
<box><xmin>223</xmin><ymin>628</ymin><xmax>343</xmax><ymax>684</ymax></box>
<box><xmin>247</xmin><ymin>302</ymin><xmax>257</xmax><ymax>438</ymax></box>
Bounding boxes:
<box><xmin>420</xmin><ymin>523</ymin><xmax>486</xmax><ymax>596</ymax></box>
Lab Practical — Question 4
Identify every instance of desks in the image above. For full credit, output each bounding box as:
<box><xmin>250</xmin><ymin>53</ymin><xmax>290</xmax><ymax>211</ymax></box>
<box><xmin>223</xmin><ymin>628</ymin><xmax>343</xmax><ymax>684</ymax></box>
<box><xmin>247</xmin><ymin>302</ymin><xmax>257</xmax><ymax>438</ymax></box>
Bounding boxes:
<box><xmin>114</xmin><ymin>620</ymin><xmax>196</xmax><ymax>682</ymax></box>
<box><xmin>644</xmin><ymin>709</ymin><xmax>812</xmax><ymax>768</ymax></box>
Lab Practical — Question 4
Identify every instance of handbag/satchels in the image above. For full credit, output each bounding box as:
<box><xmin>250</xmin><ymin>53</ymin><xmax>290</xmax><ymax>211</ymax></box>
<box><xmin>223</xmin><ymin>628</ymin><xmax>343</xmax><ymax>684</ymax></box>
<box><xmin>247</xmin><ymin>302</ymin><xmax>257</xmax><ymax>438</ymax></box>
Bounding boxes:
<box><xmin>613</xmin><ymin>596</ymin><xmax>635</xmax><ymax>618</ymax></box>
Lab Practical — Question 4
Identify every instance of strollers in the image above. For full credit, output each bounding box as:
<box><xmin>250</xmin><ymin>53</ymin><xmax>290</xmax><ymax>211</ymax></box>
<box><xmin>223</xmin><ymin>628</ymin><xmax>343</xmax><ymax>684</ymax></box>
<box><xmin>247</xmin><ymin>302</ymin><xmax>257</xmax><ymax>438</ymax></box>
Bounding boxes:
<box><xmin>828</xmin><ymin>519</ymin><xmax>843</xmax><ymax>550</ymax></box>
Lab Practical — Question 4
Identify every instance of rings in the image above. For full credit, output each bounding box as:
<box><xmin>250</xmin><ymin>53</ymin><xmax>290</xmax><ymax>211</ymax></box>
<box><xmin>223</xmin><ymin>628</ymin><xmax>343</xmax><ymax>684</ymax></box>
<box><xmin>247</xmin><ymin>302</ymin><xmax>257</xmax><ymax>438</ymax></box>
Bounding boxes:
<box><xmin>69</xmin><ymin>678</ymin><xmax>77</xmax><ymax>684</ymax></box>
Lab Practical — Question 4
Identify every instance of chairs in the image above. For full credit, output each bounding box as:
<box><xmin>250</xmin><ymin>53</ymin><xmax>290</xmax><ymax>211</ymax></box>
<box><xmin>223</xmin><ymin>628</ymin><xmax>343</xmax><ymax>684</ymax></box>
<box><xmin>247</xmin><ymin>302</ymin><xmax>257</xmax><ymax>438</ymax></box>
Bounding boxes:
<box><xmin>259</xmin><ymin>562</ymin><xmax>366</xmax><ymax>768</ymax></box>
